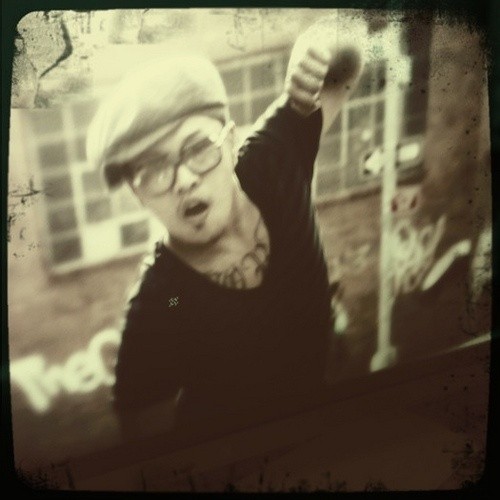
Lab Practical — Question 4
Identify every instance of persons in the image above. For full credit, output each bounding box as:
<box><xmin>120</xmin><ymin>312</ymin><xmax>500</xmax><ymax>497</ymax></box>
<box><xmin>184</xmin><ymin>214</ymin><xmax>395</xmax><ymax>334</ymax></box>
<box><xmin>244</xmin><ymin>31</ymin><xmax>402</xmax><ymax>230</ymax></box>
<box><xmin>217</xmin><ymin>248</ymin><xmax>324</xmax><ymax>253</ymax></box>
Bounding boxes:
<box><xmin>83</xmin><ymin>13</ymin><xmax>375</xmax><ymax>452</ymax></box>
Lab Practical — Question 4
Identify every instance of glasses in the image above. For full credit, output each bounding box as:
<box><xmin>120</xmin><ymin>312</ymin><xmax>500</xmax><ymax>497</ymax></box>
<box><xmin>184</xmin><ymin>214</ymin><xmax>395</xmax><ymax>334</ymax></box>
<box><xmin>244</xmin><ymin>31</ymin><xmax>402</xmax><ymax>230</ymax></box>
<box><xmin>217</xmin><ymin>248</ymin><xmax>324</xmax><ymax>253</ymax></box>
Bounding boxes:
<box><xmin>128</xmin><ymin>120</ymin><xmax>236</xmax><ymax>198</ymax></box>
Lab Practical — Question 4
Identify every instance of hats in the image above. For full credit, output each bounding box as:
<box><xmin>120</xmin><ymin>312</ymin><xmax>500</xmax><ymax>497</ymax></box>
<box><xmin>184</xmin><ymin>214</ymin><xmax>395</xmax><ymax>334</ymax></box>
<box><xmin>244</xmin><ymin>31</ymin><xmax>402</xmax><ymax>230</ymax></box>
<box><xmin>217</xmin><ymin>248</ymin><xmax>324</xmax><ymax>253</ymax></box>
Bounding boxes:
<box><xmin>86</xmin><ymin>53</ymin><xmax>229</xmax><ymax>190</ymax></box>
